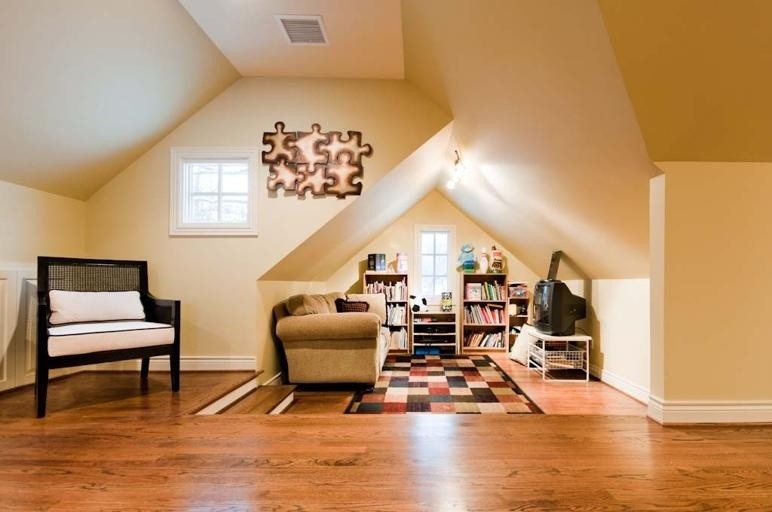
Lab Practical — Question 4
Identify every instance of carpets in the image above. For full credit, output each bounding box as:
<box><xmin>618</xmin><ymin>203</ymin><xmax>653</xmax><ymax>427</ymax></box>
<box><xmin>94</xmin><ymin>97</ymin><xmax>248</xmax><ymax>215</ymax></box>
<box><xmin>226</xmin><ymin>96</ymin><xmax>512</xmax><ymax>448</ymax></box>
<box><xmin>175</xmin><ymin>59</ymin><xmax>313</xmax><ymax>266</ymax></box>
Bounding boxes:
<box><xmin>343</xmin><ymin>355</ymin><xmax>545</xmax><ymax>414</ymax></box>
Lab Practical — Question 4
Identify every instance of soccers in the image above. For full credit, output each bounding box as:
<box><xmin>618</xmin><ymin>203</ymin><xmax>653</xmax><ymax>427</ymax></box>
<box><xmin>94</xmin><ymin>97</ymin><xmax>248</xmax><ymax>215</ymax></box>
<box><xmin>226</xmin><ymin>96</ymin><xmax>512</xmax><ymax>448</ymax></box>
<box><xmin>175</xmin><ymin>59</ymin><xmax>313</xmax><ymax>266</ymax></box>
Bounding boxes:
<box><xmin>409</xmin><ymin>294</ymin><xmax>428</xmax><ymax>314</ymax></box>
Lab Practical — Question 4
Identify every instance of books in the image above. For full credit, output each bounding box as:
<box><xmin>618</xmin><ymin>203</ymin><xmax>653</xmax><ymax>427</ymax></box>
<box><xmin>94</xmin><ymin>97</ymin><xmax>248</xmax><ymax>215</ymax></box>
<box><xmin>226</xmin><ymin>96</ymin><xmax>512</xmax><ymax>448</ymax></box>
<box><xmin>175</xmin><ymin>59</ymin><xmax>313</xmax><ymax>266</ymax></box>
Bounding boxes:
<box><xmin>366</xmin><ymin>277</ymin><xmax>408</xmax><ymax>350</ymax></box>
<box><xmin>463</xmin><ymin>280</ymin><xmax>506</xmax><ymax>348</ymax></box>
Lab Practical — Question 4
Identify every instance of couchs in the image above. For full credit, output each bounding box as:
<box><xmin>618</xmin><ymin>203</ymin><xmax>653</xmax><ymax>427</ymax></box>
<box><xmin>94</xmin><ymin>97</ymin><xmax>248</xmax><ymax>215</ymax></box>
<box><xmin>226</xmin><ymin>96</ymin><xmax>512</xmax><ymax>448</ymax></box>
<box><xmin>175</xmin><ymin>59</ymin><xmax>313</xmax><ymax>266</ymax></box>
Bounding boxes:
<box><xmin>273</xmin><ymin>293</ymin><xmax>391</xmax><ymax>393</ymax></box>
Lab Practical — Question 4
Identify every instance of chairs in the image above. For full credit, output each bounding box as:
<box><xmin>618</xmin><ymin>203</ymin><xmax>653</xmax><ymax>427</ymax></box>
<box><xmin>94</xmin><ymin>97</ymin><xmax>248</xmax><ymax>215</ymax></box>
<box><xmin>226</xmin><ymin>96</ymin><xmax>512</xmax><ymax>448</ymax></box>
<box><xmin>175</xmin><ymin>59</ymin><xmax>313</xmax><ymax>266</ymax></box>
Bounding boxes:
<box><xmin>35</xmin><ymin>256</ymin><xmax>181</xmax><ymax>418</ymax></box>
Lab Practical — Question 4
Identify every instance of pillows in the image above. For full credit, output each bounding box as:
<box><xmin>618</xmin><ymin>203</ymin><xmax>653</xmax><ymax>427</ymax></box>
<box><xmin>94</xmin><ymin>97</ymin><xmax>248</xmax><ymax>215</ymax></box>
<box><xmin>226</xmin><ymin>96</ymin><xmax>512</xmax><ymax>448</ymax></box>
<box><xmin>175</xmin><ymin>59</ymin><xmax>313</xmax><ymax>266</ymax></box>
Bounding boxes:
<box><xmin>48</xmin><ymin>289</ymin><xmax>145</xmax><ymax>325</ymax></box>
<box><xmin>335</xmin><ymin>298</ymin><xmax>369</xmax><ymax>312</ymax></box>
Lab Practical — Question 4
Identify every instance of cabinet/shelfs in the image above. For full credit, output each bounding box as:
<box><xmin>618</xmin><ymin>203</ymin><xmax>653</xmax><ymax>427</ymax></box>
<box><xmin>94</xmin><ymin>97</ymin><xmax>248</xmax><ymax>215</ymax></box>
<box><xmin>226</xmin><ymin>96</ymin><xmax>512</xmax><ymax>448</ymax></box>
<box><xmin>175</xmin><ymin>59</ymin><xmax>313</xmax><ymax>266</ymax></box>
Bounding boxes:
<box><xmin>461</xmin><ymin>273</ymin><xmax>509</xmax><ymax>355</ymax></box>
<box><xmin>526</xmin><ymin>329</ymin><xmax>592</xmax><ymax>383</ymax></box>
<box><xmin>411</xmin><ymin>312</ymin><xmax>458</xmax><ymax>356</ymax></box>
<box><xmin>508</xmin><ymin>282</ymin><xmax>532</xmax><ymax>353</ymax></box>
<box><xmin>362</xmin><ymin>272</ymin><xmax>409</xmax><ymax>355</ymax></box>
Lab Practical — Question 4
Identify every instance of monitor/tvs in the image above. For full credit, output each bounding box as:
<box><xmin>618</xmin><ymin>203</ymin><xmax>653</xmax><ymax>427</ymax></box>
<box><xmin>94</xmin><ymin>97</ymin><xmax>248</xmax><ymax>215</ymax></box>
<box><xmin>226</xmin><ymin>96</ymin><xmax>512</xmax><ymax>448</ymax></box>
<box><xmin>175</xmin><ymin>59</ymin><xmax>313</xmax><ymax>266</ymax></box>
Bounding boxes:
<box><xmin>533</xmin><ymin>282</ymin><xmax>586</xmax><ymax>336</ymax></box>
